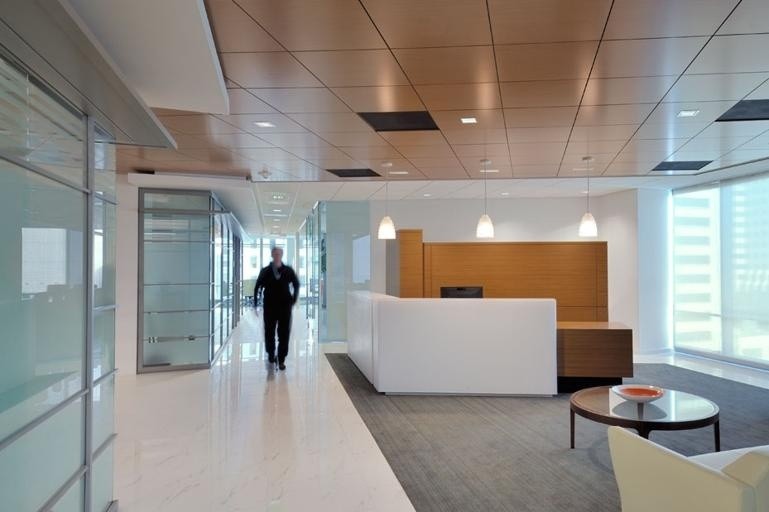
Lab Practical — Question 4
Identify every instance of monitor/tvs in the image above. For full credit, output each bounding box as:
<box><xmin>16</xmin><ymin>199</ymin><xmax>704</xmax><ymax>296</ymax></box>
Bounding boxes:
<box><xmin>439</xmin><ymin>285</ymin><xmax>483</xmax><ymax>298</ymax></box>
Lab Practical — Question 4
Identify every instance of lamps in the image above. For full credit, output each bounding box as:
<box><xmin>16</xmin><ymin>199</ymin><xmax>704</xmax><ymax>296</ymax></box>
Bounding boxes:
<box><xmin>476</xmin><ymin>159</ymin><xmax>495</xmax><ymax>239</ymax></box>
<box><xmin>376</xmin><ymin>160</ymin><xmax>396</xmax><ymax>240</ymax></box>
<box><xmin>579</xmin><ymin>156</ymin><xmax>598</xmax><ymax>237</ymax></box>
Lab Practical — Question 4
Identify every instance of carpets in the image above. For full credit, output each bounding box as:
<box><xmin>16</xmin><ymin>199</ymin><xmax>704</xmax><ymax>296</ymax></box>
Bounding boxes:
<box><xmin>321</xmin><ymin>352</ymin><xmax>769</xmax><ymax>512</ymax></box>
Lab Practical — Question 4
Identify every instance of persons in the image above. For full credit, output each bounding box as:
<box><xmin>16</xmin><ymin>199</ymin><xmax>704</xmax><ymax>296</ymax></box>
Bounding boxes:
<box><xmin>253</xmin><ymin>248</ymin><xmax>301</xmax><ymax>371</ymax></box>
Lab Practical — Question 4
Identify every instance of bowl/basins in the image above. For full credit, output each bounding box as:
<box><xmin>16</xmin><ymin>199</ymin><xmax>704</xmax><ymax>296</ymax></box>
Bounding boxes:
<box><xmin>607</xmin><ymin>401</ymin><xmax>668</xmax><ymax>420</ymax></box>
<box><xmin>611</xmin><ymin>384</ymin><xmax>667</xmax><ymax>403</ymax></box>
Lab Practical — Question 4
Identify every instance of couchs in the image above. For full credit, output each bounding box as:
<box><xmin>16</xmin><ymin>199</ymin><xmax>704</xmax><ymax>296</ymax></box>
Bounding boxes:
<box><xmin>607</xmin><ymin>425</ymin><xmax>769</xmax><ymax>512</ymax></box>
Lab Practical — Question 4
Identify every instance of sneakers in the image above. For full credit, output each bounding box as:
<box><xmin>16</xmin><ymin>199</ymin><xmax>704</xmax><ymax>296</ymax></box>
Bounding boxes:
<box><xmin>267</xmin><ymin>351</ymin><xmax>289</xmax><ymax>372</ymax></box>
